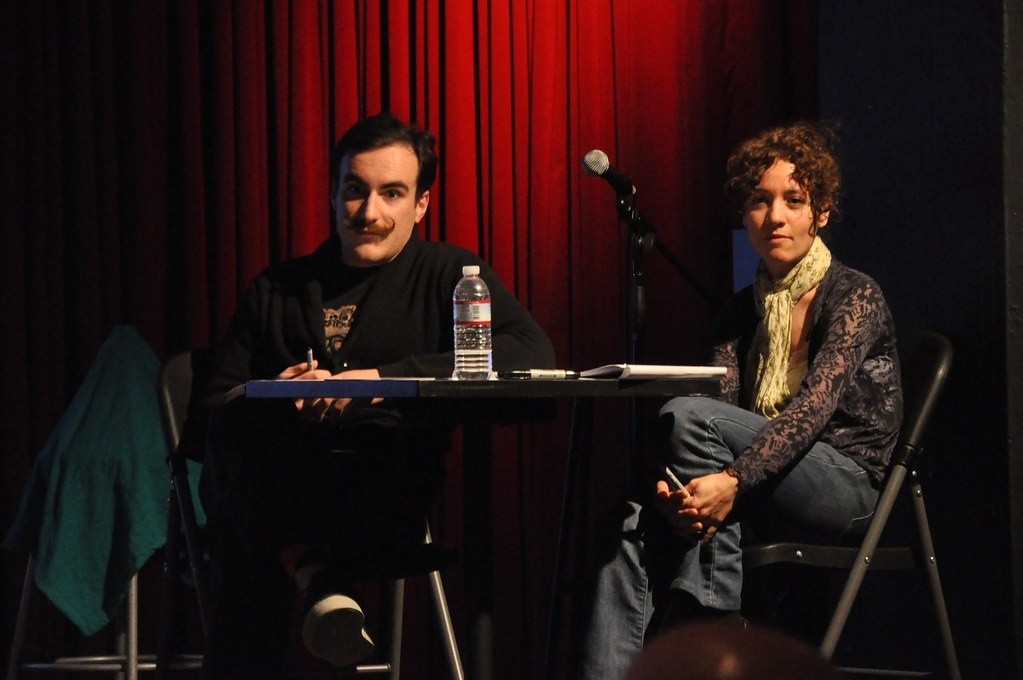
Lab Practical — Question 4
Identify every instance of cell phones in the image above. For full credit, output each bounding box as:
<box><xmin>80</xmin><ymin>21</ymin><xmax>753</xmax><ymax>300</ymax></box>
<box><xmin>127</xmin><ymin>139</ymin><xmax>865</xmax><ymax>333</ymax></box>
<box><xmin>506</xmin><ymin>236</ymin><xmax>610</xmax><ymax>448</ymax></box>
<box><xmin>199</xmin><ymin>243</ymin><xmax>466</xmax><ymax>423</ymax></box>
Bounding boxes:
<box><xmin>498</xmin><ymin>371</ymin><xmax>580</xmax><ymax>380</ymax></box>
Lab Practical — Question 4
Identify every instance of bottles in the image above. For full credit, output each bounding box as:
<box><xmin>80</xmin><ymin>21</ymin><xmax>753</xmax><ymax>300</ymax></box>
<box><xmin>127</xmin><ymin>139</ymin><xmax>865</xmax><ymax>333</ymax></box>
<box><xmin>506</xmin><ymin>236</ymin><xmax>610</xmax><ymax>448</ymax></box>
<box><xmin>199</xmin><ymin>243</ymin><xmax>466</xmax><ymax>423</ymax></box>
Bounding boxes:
<box><xmin>453</xmin><ymin>265</ymin><xmax>492</xmax><ymax>381</ymax></box>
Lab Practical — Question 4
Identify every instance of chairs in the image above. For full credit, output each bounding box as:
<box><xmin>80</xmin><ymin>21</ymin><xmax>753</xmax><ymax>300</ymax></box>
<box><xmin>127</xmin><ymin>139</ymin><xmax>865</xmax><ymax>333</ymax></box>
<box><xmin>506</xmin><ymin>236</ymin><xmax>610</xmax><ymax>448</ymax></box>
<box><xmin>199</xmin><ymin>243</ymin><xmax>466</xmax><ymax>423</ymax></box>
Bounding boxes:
<box><xmin>740</xmin><ymin>328</ymin><xmax>962</xmax><ymax>680</ymax></box>
<box><xmin>158</xmin><ymin>346</ymin><xmax>466</xmax><ymax>680</ymax></box>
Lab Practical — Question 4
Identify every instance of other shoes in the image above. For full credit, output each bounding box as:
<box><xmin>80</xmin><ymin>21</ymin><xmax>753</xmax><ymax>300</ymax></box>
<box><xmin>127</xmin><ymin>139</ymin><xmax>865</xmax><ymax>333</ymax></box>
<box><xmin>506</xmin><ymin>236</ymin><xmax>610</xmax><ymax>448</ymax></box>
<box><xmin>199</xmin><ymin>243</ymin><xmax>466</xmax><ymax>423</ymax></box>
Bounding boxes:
<box><xmin>303</xmin><ymin>593</ymin><xmax>375</xmax><ymax>669</ymax></box>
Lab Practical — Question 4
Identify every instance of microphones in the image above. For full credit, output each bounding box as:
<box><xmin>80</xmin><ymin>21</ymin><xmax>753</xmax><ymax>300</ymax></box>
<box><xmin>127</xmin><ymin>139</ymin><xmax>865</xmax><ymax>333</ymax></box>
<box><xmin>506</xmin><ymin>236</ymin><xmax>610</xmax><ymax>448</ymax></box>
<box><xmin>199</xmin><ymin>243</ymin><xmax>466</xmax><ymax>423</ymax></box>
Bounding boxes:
<box><xmin>582</xmin><ymin>150</ymin><xmax>638</xmax><ymax>200</ymax></box>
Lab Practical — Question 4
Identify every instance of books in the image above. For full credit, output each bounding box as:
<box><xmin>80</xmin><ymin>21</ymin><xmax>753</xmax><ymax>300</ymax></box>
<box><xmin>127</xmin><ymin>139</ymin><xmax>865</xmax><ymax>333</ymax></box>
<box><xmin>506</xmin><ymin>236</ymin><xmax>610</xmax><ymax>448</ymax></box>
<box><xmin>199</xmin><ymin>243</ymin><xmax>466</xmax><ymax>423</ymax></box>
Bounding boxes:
<box><xmin>580</xmin><ymin>363</ymin><xmax>728</xmax><ymax>377</ymax></box>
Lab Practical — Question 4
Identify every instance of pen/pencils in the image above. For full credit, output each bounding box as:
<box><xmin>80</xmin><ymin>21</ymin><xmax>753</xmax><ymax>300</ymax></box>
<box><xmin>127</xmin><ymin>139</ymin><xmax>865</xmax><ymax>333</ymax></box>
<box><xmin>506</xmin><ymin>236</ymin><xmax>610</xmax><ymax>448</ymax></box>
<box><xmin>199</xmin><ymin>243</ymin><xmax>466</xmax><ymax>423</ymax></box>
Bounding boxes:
<box><xmin>307</xmin><ymin>347</ymin><xmax>313</xmax><ymax>371</ymax></box>
<box><xmin>665</xmin><ymin>466</ymin><xmax>691</xmax><ymax>498</ymax></box>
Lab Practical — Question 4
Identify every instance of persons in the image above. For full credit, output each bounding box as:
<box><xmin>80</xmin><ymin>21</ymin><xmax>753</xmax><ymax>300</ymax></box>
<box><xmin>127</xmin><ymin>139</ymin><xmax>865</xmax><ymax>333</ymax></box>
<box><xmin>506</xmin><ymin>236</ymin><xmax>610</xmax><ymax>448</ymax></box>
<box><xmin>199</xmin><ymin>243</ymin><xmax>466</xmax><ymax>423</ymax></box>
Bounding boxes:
<box><xmin>581</xmin><ymin>126</ymin><xmax>905</xmax><ymax>680</ymax></box>
<box><xmin>186</xmin><ymin>116</ymin><xmax>556</xmax><ymax>680</ymax></box>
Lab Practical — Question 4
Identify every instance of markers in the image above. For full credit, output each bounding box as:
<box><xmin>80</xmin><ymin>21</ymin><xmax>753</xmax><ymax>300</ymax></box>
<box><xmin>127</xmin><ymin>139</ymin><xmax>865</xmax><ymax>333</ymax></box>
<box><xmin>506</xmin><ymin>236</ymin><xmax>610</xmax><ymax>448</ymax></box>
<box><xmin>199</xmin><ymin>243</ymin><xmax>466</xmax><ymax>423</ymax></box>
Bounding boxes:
<box><xmin>498</xmin><ymin>370</ymin><xmax>580</xmax><ymax>380</ymax></box>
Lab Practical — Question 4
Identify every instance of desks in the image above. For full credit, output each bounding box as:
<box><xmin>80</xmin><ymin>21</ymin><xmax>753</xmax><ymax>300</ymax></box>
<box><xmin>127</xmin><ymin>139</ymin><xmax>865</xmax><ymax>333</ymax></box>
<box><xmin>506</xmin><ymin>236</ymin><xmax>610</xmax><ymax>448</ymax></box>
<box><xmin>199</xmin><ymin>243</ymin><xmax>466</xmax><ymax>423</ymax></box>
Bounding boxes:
<box><xmin>202</xmin><ymin>373</ymin><xmax>723</xmax><ymax>680</ymax></box>
